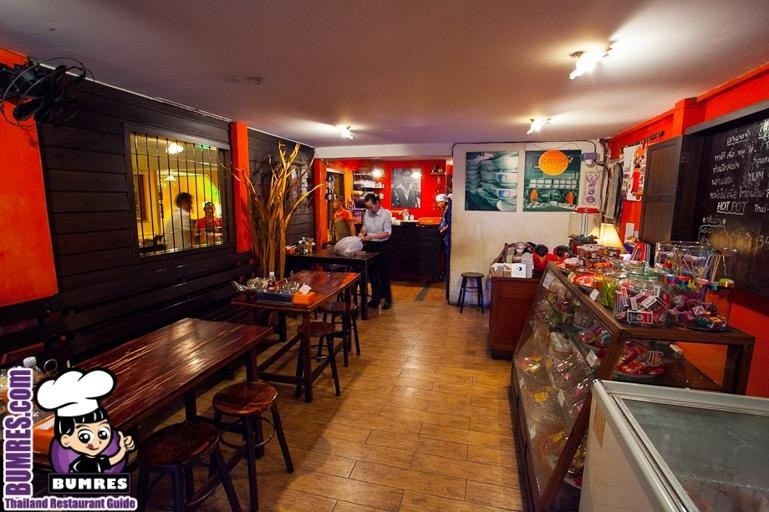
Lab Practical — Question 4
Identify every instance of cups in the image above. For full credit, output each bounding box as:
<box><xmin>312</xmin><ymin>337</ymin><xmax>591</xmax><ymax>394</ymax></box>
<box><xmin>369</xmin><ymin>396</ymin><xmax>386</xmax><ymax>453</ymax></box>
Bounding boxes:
<box><xmin>278</xmin><ymin>271</ymin><xmax>300</xmax><ymax>294</ymax></box>
<box><xmin>410</xmin><ymin>215</ymin><xmax>414</xmax><ymax>220</ymax></box>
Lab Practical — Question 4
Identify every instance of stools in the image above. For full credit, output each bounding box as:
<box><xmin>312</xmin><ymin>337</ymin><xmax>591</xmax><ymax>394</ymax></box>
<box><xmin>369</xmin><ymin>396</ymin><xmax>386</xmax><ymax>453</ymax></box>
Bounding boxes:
<box><xmin>455</xmin><ymin>272</ymin><xmax>485</xmax><ymax>314</ymax></box>
<box><xmin>297</xmin><ymin>320</ymin><xmax>341</xmax><ymax>401</ymax></box>
<box><xmin>209</xmin><ymin>380</ymin><xmax>294</xmax><ymax>512</ymax></box>
<box><xmin>319</xmin><ymin>302</ymin><xmax>361</xmax><ymax>367</ymax></box>
<box><xmin>134</xmin><ymin>419</ymin><xmax>241</xmax><ymax>512</ymax></box>
<box><xmin>334</xmin><ymin>270</ymin><xmax>359</xmax><ymax>306</ymax></box>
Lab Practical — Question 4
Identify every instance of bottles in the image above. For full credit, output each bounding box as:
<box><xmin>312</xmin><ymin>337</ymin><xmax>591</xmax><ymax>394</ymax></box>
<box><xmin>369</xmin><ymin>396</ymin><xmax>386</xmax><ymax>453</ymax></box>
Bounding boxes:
<box><xmin>358</xmin><ymin>179</ymin><xmax>382</xmax><ymax>188</ymax></box>
<box><xmin>301</xmin><ymin>246</ymin><xmax>305</xmax><ymax>254</ymax></box>
<box><xmin>268</xmin><ymin>271</ymin><xmax>276</xmax><ymax>287</ymax></box>
<box><xmin>354</xmin><ymin>167</ymin><xmax>384</xmax><ymax>175</ymax></box>
<box><xmin>23</xmin><ymin>356</ymin><xmax>47</xmax><ymax>417</ymax></box>
<box><xmin>405</xmin><ymin>214</ymin><xmax>408</xmax><ymax>220</ymax></box>
<box><xmin>564</xmin><ymin>240</ymin><xmax>739</xmax><ymax>332</ymax></box>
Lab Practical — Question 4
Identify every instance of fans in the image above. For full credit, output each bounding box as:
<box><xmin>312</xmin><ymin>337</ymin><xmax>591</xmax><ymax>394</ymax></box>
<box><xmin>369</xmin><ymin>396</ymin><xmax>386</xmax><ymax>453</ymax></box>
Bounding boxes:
<box><xmin>0</xmin><ymin>55</ymin><xmax>97</xmax><ymax>132</ymax></box>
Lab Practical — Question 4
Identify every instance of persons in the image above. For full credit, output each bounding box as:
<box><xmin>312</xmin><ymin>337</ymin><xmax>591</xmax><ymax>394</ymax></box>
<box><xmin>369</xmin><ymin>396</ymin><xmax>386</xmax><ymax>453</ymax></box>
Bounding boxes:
<box><xmin>332</xmin><ymin>195</ymin><xmax>356</xmax><ymax>241</ymax></box>
<box><xmin>359</xmin><ymin>193</ymin><xmax>393</xmax><ymax>309</ymax></box>
<box><xmin>393</xmin><ymin>168</ymin><xmax>420</xmax><ymax>208</ymax></box>
<box><xmin>436</xmin><ymin>192</ymin><xmax>449</xmax><ymax>248</ymax></box>
<box><xmin>162</xmin><ymin>192</ymin><xmax>195</xmax><ymax>249</ymax></box>
<box><xmin>195</xmin><ymin>201</ymin><xmax>222</xmax><ymax>244</ymax></box>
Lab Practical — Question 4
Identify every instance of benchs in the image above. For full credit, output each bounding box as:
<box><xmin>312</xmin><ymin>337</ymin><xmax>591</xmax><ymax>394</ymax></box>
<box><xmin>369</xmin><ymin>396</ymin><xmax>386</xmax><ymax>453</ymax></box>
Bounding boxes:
<box><xmin>0</xmin><ymin>249</ymin><xmax>285</xmax><ymax>437</ymax></box>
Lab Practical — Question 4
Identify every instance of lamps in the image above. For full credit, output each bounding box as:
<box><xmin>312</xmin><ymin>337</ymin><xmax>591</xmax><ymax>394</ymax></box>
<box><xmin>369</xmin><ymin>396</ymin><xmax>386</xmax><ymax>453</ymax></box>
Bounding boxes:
<box><xmin>589</xmin><ymin>223</ymin><xmax>627</xmax><ymax>253</ymax></box>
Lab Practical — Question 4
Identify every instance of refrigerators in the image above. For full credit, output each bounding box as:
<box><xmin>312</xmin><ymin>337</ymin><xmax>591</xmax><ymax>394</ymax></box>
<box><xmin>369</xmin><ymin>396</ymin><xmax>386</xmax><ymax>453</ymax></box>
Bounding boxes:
<box><xmin>576</xmin><ymin>380</ymin><xmax>768</xmax><ymax>512</ymax></box>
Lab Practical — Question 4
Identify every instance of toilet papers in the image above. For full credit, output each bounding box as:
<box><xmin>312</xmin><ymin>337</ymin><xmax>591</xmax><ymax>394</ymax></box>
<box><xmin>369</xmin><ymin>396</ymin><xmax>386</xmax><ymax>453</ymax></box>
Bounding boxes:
<box><xmin>291</xmin><ymin>284</ymin><xmax>316</xmax><ymax>306</ymax></box>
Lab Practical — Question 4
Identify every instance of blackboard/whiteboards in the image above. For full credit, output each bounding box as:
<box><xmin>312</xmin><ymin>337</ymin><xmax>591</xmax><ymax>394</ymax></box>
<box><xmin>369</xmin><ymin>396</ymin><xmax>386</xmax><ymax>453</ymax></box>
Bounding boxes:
<box><xmin>690</xmin><ymin>111</ymin><xmax>769</xmax><ymax>297</ymax></box>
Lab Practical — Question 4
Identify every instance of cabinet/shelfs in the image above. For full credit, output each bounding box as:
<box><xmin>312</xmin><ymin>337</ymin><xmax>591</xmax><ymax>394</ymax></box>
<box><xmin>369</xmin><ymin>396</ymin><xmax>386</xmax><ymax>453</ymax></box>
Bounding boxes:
<box><xmin>352</xmin><ymin>171</ymin><xmax>385</xmax><ymax>211</ymax></box>
<box><xmin>489</xmin><ymin>275</ymin><xmax>538</xmax><ymax>359</ymax></box>
<box><xmin>508</xmin><ymin>261</ymin><xmax>756</xmax><ymax>511</ymax></box>
